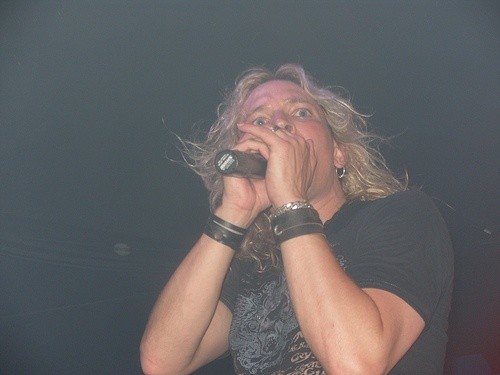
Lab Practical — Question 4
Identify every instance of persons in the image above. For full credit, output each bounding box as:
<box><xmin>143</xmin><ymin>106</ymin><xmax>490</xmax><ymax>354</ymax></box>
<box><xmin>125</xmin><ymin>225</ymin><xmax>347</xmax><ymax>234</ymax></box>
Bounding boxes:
<box><xmin>140</xmin><ymin>64</ymin><xmax>455</xmax><ymax>375</ymax></box>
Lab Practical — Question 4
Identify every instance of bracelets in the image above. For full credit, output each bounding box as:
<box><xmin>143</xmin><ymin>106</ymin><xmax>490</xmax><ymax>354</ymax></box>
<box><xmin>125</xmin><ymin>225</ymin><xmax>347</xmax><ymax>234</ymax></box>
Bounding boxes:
<box><xmin>202</xmin><ymin>213</ymin><xmax>249</xmax><ymax>252</ymax></box>
<box><xmin>267</xmin><ymin>200</ymin><xmax>329</xmax><ymax>250</ymax></box>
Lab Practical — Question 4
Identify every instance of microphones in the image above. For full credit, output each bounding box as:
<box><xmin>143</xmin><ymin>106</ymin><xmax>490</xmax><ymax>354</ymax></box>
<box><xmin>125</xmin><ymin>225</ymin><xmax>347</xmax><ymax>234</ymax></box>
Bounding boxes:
<box><xmin>214</xmin><ymin>150</ymin><xmax>268</xmax><ymax>179</ymax></box>
